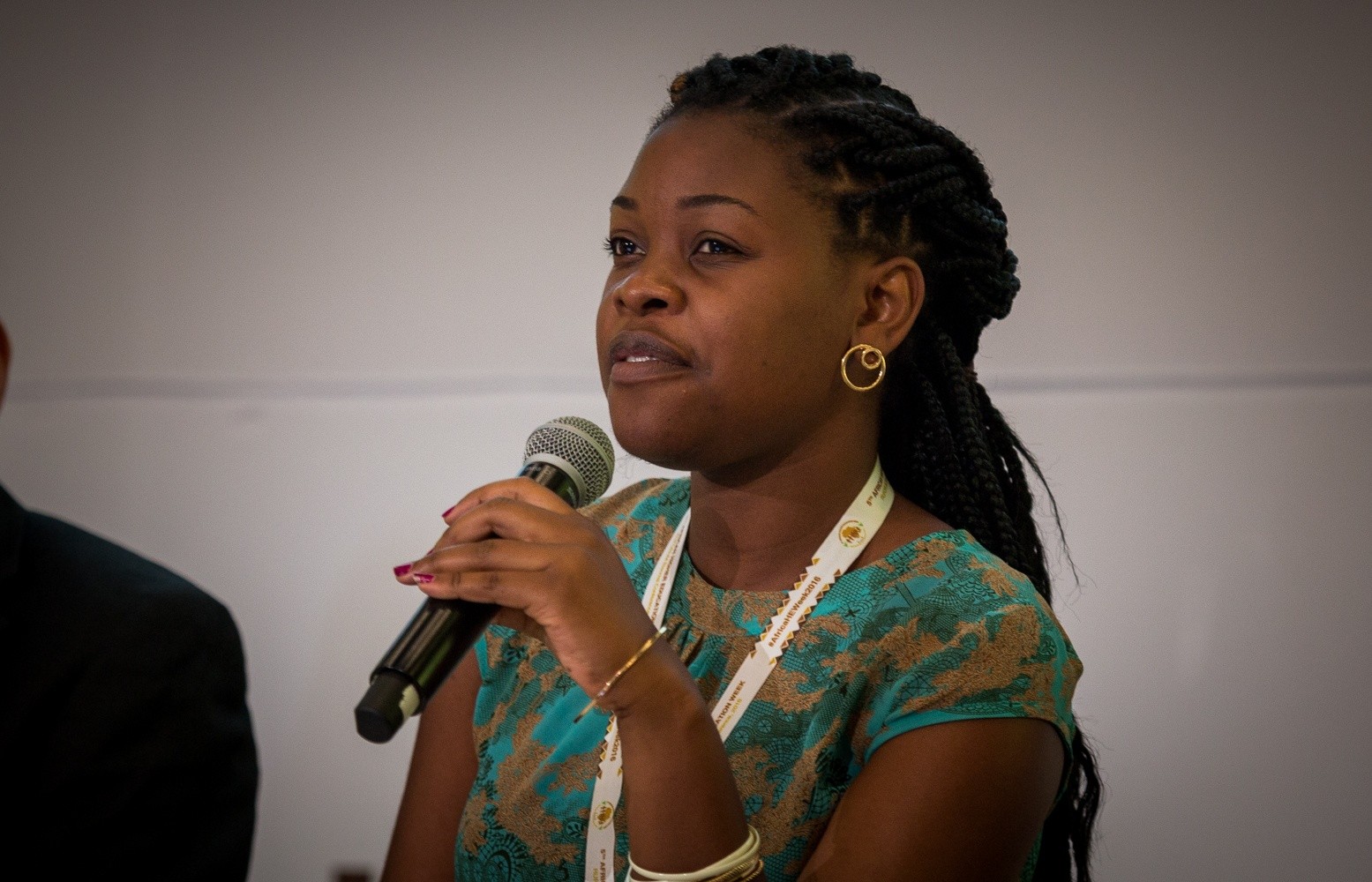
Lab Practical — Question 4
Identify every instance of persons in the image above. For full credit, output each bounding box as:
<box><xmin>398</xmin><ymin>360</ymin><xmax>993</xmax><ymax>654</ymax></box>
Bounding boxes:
<box><xmin>326</xmin><ymin>40</ymin><xmax>1092</xmax><ymax>881</ymax></box>
<box><xmin>0</xmin><ymin>325</ymin><xmax>264</xmax><ymax>882</ymax></box>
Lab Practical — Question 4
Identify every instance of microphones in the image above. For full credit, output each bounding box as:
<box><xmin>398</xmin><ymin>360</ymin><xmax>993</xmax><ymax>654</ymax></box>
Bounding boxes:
<box><xmin>355</xmin><ymin>415</ymin><xmax>614</xmax><ymax>745</ymax></box>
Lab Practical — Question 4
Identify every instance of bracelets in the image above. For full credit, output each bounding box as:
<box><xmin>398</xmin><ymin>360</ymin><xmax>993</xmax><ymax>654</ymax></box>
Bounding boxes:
<box><xmin>616</xmin><ymin>823</ymin><xmax>768</xmax><ymax>882</ymax></box>
<box><xmin>569</xmin><ymin>611</ymin><xmax>687</xmax><ymax>727</ymax></box>
